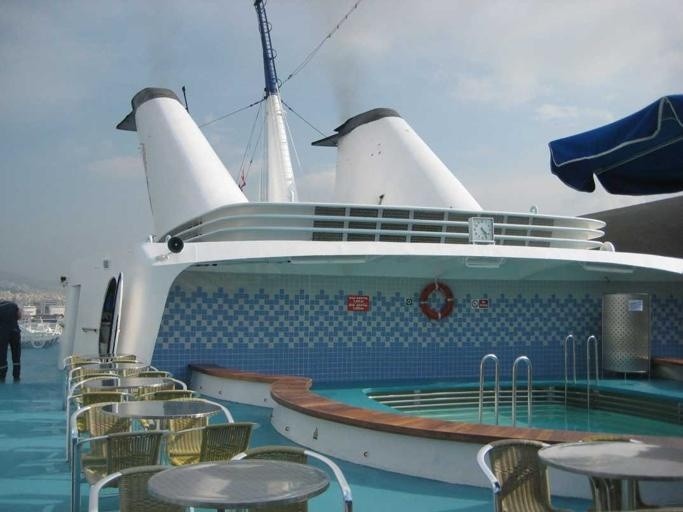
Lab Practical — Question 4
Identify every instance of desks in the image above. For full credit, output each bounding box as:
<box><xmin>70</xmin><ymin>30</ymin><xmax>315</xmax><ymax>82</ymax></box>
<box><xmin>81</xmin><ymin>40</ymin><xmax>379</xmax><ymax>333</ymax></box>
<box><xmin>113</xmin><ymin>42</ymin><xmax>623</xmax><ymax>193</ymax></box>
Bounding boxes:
<box><xmin>536</xmin><ymin>440</ymin><xmax>683</xmax><ymax>482</ymax></box>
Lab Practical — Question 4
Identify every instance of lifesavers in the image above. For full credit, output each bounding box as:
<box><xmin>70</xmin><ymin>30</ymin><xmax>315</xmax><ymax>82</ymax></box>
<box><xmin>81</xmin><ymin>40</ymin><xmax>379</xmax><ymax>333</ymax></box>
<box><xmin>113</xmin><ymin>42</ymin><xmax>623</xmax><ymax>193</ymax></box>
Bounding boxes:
<box><xmin>420</xmin><ymin>283</ymin><xmax>453</xmax><ymax>320</ymax></box>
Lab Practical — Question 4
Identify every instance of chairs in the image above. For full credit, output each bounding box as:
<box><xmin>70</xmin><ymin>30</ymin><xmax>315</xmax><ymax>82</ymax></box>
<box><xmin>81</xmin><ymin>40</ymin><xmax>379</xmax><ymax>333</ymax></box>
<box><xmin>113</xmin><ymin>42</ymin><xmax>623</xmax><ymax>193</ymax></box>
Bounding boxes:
<box><xmin>476</xmin><ymin>438</ymin><xmax>573</xmax><ymax>512</ymax></box>
<box><xmin>576</xmin><ymin>434</ymin><xmax>683</xmax><ymax>512</ymax></box>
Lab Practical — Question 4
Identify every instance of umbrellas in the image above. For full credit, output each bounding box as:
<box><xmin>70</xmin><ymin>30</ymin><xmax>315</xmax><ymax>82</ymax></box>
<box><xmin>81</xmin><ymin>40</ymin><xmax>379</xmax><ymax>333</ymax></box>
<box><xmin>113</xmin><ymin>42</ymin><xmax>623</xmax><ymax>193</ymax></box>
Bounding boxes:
<box><xmin>548</xmin><ymin>94</ymin><xmax>682</xmax><ymax>195</ymax></box>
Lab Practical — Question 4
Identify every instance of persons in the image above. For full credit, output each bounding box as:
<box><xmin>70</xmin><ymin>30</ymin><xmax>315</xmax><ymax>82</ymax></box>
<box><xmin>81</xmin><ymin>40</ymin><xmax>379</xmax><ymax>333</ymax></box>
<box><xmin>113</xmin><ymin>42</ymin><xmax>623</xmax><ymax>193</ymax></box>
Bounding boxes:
<box><xmin>0</xmin><ymin>297</ymin><xmax>23</xmax><ymax>382</ymax></box>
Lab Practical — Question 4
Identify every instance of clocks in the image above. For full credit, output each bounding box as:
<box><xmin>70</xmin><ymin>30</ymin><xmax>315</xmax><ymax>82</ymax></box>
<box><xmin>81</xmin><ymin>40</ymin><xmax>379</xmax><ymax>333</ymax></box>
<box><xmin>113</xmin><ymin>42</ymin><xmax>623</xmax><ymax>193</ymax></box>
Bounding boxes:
<box><xmin>468</xmin><ymin>216</ymin><xmax>496</xmax><ymax>245</ymax></box>
